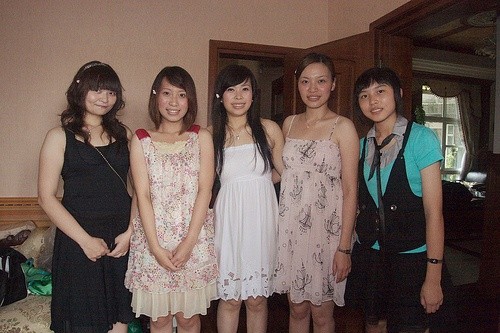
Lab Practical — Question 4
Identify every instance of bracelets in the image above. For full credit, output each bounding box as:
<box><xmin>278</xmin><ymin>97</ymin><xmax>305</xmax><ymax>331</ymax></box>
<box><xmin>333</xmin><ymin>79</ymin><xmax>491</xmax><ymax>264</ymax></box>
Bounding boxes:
<box><xmin>336</xmin><ymin>246</ymin><xmax>351</xmax><ymax>255</ymax></box>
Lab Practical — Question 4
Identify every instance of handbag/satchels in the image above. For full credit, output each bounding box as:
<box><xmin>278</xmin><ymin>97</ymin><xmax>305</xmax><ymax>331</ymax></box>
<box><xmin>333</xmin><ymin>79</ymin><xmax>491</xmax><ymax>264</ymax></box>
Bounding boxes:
<box><xmin>343</xmin><ymin>241</ymin><xmax>389</xmax><ymax>306</ymax></box>
<box><xmin>19</xmin><ymin>256</ymin><xmax>54</xmax><ymax>297</ymax></box>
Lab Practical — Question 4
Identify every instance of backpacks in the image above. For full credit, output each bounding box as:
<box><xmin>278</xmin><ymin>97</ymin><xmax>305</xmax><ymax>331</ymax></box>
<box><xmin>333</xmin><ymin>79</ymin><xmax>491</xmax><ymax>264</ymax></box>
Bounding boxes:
<box><xmin>0</xmin><ymin>237</ymin><xmax>36</xmax><ymax>308</ymax></box>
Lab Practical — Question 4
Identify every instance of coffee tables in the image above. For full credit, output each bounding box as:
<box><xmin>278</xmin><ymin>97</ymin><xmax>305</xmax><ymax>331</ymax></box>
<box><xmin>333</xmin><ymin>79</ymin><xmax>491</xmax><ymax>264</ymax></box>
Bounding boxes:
<box><xmin>470</xmin><ymin>189</ymin><xmax>486</xmax><ymax>199</ymax></box>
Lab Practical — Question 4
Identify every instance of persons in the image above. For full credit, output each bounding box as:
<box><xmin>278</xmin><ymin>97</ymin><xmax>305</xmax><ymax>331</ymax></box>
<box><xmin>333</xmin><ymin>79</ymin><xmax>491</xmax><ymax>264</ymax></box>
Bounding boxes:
<box><xmin>130</xmin><ymin>65</ymin><xmax>221</xmax><ymax>333</ymax></box>
<box><xmin>333</xmin><ymin>67</ymin><xmax>445</xmax><ymax>333</ymax></box>
<box><xmin>200</xmin><ymin>65</ymin><xmax>291</xmax><ymax>333</ymax></box>
<box><xmin>37</xmin><ymin>60</ymin><xmax>143</xmax><ymax>333</ymax></box>
<box><xmin>267</xmin><ymin>51</ymin><xmax>363</xmax><ymax>332</ymax></box>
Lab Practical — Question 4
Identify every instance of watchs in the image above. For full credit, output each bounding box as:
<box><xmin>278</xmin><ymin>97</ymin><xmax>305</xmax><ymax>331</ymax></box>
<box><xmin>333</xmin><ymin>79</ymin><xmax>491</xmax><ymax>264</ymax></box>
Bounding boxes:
<box><xmin>425</xmin><ymin>257</ymin><xmax>444</xmax><ymax>264</ymax></box>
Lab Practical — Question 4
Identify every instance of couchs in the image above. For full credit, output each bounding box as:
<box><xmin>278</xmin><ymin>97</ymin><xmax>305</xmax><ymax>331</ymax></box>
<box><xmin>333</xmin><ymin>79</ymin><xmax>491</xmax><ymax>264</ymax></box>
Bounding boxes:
<box><xmin>0</xmin><ymin>219</ymin><xmax>144</xmax><ymax>333</ymax></box>
<box><xmin>442</xmin><ymin>180</ymin><xmax>484</xmax><ymax>248</ymax></box>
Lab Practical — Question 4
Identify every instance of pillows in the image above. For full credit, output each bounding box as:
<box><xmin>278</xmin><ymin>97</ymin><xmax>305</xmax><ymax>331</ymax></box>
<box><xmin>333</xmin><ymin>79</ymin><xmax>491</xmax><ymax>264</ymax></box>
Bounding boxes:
<box><xmin>467</xmin><ymin>172</ymin><xmax>487</xmax><ymax>182</ymax></box>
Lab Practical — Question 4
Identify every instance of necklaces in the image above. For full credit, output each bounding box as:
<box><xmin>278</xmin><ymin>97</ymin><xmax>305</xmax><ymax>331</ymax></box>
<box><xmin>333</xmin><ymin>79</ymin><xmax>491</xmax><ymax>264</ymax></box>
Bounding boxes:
<box><xmin>226</xmin><ymin>117</ymin><xmax>247</xmax><ymax>140</ymax></box>
<box><xmin>80</xmin><ymin>122</ymin><xmax>102</xmax><ymax>136</ymax></box>
<box><xmin>304</xmin><ymin>110</ymin><xmax>330</xmax><ymax>129</ymax></box>
